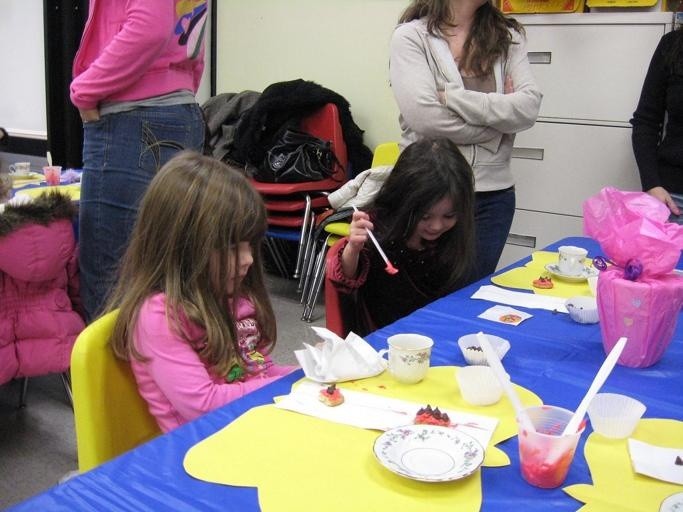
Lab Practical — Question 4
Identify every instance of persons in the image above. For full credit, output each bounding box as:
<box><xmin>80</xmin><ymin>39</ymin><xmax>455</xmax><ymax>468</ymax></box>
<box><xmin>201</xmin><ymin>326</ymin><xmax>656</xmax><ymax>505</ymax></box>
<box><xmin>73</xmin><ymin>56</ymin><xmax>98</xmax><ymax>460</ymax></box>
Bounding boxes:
<box><xmin>70</xmin><ymin>0</ymin><xmax>212</xmax><ymax>328</ymax></box>
<box><xmin>101</xmin><ymin>149</ymin><xmax>302</xmax><ymax>433</ymax></box>
<box><xmin>390</xmin><ymin>0</ymin><xmax>543</xmax><ymax>279</ymax></box>
<box><xmin>631</xmin><ymin>24</ymin><xmax>683</xmax><ymax>220</ymax></box>
<box><xmin>325</xmin><ymin>136</ymin><xmax>483</xmax><ymax>337</ymax></box>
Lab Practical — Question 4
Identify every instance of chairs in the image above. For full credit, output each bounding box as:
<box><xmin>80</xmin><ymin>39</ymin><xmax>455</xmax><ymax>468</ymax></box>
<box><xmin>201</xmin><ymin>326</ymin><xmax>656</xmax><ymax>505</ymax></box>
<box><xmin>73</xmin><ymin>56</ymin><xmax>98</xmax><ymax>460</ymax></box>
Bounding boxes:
<box><xmin>300</xmin><ymin>141</ymin><xmax>405</xmax><ymax>323</ymax></box>
<box><xmin>0</xmin><ymin>195</ymin><xmax>86</xmax><ymax>417</ymax></box>
<box><xmin>65</xmin><ymin>306</ymin><xmax>158</xmax><ymax>477</ymax></box>
<box><xmin>246</xmin><ymin>102</ymin><xmax>354</xmax><ymax>302</ymax></box>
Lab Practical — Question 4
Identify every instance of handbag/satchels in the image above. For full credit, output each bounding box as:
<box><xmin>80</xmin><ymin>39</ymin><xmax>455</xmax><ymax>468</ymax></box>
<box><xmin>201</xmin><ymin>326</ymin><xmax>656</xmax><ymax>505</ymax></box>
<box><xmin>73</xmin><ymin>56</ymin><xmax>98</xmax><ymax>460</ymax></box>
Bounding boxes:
<box><xmin>253</xmin><ymin>129</ymin><xmax>333</xmax><ymax>182</ymax></box>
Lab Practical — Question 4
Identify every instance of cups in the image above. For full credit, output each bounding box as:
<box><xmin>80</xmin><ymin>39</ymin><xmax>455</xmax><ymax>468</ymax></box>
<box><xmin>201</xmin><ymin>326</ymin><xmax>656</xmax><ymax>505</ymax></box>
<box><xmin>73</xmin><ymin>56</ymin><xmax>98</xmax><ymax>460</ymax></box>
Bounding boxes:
<box><xmin>377</xmin><ymin>333</ymin><xmax>433</xmax><ymax>386</ymax></box>
<box><xmin>7</xmin><ymin>161</ymin><xmax>30</xmax><ymax>175</ymax></box>
<box><xmin>455</xmin><ymin>363</ymin><xmax>510</xmax><ymax>408</ymax></box>
<box><xmin>586</xmin><ymin>392</ymin><xmax>648</xmax><ymax>442</ymax></box>
<box><xmin>457</xmin><ymin>332</ymin><xmax>507</xmax><ymax>371</ymax></box>
<box><xmin>557</xmin><ymin>244</ymin><xmax>588</xmax><ymax>275</ymax></box>
<box><xmin>516</xmin><ymin>406</ymin><xmax>588</xmax><ymax>491</ymax></box>
<box><xmin>566</xmin><ymin>296</ymin><xmax>600</xmax><ymax>323</ymax></box>
<box><xmin>43</xmin><ymin>166</ymin><xmax>62</xmax><ymax>187</ymax></box>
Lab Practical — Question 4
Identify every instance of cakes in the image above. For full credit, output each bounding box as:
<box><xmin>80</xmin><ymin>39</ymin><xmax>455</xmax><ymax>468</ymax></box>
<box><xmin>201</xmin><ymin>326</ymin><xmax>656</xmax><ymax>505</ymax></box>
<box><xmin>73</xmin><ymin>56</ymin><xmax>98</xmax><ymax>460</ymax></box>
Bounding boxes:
<box><xmin>319</xmin><ymin>383</ymin><xmax>344</xmax><ymax>407</ymax></box>
<box><xmin>415</xmin><ymin>404</ymin><xmax>451</xmax><ymax>427</ymax></box>
<box><xmin>533</xmin><ymin>275</ymin><xmax>553</xmax><ymax>289</ymax></box>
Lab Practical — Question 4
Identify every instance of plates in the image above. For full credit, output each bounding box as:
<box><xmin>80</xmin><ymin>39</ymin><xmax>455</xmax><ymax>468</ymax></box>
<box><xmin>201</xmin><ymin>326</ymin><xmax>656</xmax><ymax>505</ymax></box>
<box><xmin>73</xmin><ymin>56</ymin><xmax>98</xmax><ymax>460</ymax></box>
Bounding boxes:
<box><xmin>371</xmin><ymin>424</ymin><xmax>484</xmax><ymax>486</ymax></box>
<box><xmin>545</xmin><ymin>262</ymin><xmax>599</xmax><ymax>285</ymax></box>
<box><xmin>9</xmin><ymin>172</ymin><xmax>39</xmax><ymax>180</ymax></box>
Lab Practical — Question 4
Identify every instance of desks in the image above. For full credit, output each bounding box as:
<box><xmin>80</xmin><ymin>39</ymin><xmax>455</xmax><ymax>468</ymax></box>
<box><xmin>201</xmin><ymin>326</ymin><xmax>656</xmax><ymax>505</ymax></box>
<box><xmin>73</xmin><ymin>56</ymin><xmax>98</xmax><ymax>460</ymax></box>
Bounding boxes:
<box><xmin>0</xmin><ymin>139</ymin><xmax>682</xmax><ymax>511</ymax></box>
<box><xmin>0</xmin><ymin>151</ymin><xmax>85</xmax><ymax>220</ymax></box>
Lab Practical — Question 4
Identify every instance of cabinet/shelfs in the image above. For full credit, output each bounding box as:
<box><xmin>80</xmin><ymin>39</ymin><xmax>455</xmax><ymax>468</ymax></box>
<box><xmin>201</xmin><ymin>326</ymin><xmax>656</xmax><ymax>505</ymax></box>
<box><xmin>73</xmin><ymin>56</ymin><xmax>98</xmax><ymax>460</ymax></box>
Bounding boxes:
<box><xmin>483</xmin><ymin>10</ymin><xmax>683</xmax><ymax>278</ymax></box>
